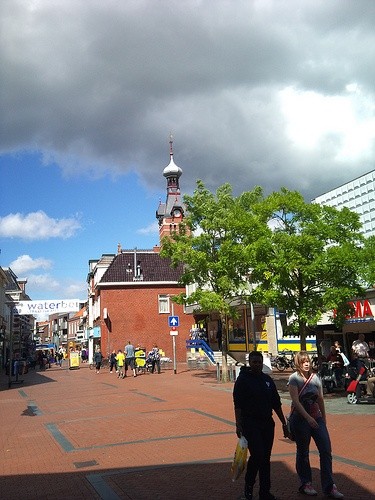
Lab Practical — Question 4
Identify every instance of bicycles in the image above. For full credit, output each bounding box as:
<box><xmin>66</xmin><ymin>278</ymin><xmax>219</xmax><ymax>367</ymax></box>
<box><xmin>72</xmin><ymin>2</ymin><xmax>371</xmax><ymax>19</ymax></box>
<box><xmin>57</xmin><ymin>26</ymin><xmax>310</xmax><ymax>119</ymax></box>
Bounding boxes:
<box><xmin>275</xmin><ymin>350</ymin><xmax>296</xmax><ymax>372</ymax></box>
<box><xmin>310</xmin><ymin>353</ymin><xmax>319</xmax><ymax>371</ymax></box>
<box><xmin>89</xmin><ymin>357</ymin><xmax>104</xmax><ymax>370</ymax></box>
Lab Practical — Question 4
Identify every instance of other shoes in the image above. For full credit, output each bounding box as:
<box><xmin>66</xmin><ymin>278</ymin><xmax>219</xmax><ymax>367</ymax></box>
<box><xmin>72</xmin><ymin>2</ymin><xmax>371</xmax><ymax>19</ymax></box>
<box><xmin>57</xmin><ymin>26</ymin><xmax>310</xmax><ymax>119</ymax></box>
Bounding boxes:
<box><xmin>259</xmin><ymin>493</ymin><xmax>280</xmax><ymax>500</ymax></box>
<box><xmin>302</xmin><ymin>481</ymin><xmax>318</xmax><ymax>496</ymax></box>
<box><xmin>240</xmin><ymin>495</ymin><xmax>252</xmax><ymax>500</ymax></box>
<box><xmin>330</xmin><ymin>483</ymin><xmax>344</xmax><ymax>498</ymax></box>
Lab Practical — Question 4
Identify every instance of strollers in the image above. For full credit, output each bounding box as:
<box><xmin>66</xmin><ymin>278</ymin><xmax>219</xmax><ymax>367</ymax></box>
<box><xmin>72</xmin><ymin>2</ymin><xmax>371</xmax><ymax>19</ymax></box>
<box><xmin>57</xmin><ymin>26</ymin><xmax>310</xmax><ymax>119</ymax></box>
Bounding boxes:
<box><xmin>145</xmin><ymin>352</ymin><xmax>156</xmax><ymax>372</ymax></box>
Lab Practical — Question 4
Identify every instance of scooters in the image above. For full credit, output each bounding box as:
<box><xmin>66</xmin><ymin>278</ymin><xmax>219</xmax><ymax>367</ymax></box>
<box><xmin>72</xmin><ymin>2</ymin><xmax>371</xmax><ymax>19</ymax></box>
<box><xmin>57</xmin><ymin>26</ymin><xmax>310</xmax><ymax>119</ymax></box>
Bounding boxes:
<box><xmin>320</xmin><ymin>360</ymin><xmax>375</xmax><ymax>404</ymax></box>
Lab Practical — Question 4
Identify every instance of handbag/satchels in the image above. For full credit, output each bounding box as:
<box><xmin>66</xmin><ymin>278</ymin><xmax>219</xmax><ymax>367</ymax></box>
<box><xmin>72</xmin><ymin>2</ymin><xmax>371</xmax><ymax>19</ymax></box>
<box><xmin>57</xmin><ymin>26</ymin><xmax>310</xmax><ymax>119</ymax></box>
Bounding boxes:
<box><xmin>230</xmin><ymin>432</ymin><xmax>248</xmax><ymax>484</ymax></box>
<box><xmin>286</xmin><ymin>414</ymin><xmax>295</xmax><ymax>441</ymax></box>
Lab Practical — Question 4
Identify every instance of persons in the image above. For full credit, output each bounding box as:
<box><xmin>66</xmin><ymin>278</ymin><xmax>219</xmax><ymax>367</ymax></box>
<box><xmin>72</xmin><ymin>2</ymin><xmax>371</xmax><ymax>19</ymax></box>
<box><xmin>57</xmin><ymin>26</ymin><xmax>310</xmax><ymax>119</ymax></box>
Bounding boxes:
<box><xmin>94</xmin><ymin>348</ymin><xmax>102</xmax><ymax>373</ymax></box>
<box><xmin>79</xmin><ymin>347</ymin><xmax>89</xmax><ymax>363</ymax></box>
<box><xmin>29</xmin><ymin>352</ymin><xmax>50</xmax><ymax>370</ymax></box>
<box><xmin>312</xmin><ymin>340</ymin><xmax>375</xmax><ymax>398</ymax></box>
<box><xmin>216</xmin><ymin>327</ymin><xmax>222</xmax><ymax>351</ymax></box>
<box><xmin>123</xmin><ymin>340</ymin><xmax>136</xmax><ymax>377</ymax></box>
<box><xmin>109</xmin><ymin>349</ymin><xmax>117</xmax><ymax>373</ymax></box>
<box><xmin>352</xmin><ymin>334</ymin><xmax>370</xmax><ymax>369</ymax></box>
<box><xmin>135</xmin><ymin>343</ymin><xmax>140</xmax><ymax>349</ymax></box>
<box><xmin>233</xmin><ymin>351</ymin><xmax>290</xmax><ymax>500</ymax></box>
<box><xmin>55</xmin><ymin>350</ymin><xmax>67</xmax><ymax>368</ymax></box>
<box><xmin>287</xmin><ymin>350</ymin><xmax>343</xmax><ymax>500</ymax></box>
<box><xmin>114</xmin><ymin>350</ymin><xmax>126</xmax><ymax>379</ymax></box>
<box><xmin>150</xmin><ymin>343</ymin><xmax>160</xmax><ymax>373</ymax></box>
